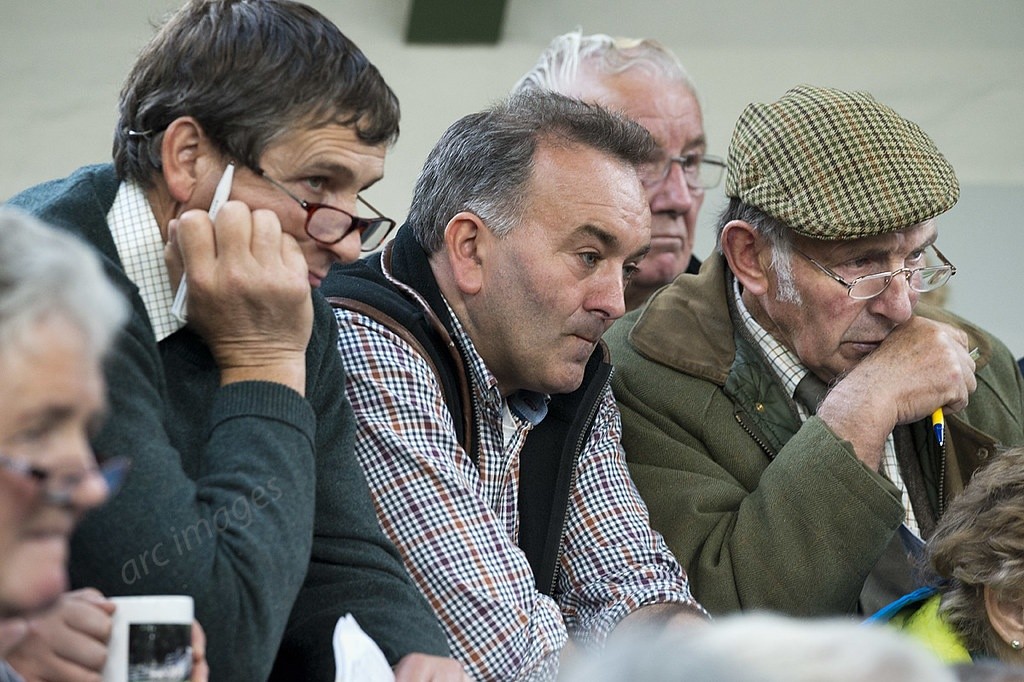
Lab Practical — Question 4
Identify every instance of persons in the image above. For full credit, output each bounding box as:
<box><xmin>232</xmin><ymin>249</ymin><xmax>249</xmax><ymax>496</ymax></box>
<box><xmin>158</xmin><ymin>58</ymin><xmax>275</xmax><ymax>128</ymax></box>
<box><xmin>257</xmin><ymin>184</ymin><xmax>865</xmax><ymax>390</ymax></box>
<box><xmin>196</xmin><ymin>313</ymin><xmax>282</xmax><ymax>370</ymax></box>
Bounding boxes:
<box><xmin>507</xmin><ymin>31</ymin><xmax>731</xmax><ymax>288</ymax></box>
<box><xmin>0</xmin><ymin>1</ymin><xmax>470</xmax><ymax>682</ymax></box>
<box><xmin>0</xmin><ymin>87</ymin><xmax>1024</xmax><ymax>681</ymax></box>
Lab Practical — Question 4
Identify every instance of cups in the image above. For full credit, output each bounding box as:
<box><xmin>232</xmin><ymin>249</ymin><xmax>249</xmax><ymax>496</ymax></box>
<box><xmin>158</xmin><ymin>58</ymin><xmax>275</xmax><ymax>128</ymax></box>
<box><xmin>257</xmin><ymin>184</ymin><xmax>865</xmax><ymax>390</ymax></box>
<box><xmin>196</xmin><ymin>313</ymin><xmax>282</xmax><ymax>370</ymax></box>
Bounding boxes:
<box><xmin>101</xmin><ymin>595</ymin><xmax>191</xmax><ymax>682</ymax></box>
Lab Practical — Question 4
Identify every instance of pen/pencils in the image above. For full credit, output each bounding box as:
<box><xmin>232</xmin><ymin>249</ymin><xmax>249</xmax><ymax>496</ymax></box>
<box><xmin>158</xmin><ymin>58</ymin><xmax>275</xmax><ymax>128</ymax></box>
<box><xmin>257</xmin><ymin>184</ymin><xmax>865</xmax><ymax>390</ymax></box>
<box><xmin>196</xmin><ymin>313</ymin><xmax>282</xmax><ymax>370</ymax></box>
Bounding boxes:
<box><xmin>933</xmin><ymin>404</ymin><xmax>944</xmax><ymax>447</ymax></box>
<box><xmin>172</xmin><ymin>160</ymin><xmax>236</xmax><ymax>325</ymax></box>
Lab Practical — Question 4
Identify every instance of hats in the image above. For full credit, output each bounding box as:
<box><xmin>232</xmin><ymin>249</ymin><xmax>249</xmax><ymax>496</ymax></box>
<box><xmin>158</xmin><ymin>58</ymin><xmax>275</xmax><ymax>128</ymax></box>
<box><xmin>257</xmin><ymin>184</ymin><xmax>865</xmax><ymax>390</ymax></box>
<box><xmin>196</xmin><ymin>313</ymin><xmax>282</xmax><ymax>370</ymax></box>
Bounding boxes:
<box><xmin>726</xmin><ymin>83</ymin><xmax>960</xmax><ymax>240</ymax></box>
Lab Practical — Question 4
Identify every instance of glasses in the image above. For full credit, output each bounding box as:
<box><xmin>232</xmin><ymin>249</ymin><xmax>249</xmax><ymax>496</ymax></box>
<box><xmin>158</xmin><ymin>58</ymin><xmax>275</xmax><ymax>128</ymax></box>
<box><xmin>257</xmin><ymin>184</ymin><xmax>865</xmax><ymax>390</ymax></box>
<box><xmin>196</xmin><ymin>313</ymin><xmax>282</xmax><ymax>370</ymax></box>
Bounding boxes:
<box><xmin>778</xmin><ymin>233</ymin><xmax>960</xmax><ymax>301</ymax></box>
<box><xmin>0</xmin><ymin>455</ymin><xmax>133</xmax><ymax>514</ymax></box>
<box><xmin>235</xmin><ymin>153</ymin><xmax>397</xmax><ymax>252</ymax></box>
<box><xmin>633</xmin><ymin>153</ymin><xmax>727</xmax><ymax>190</ymax></box>
